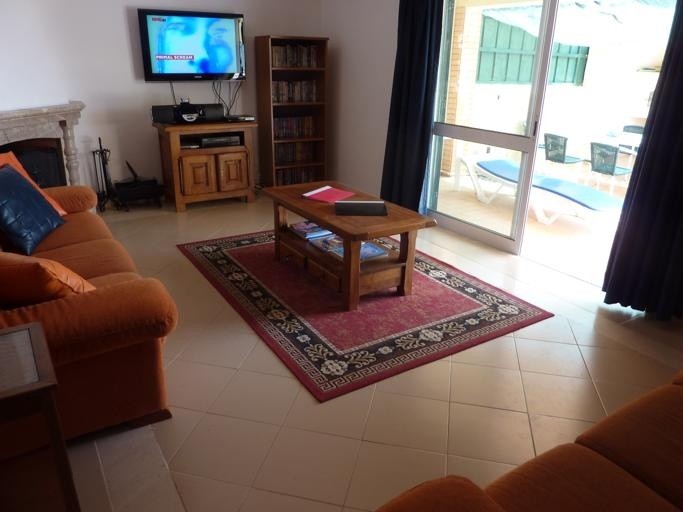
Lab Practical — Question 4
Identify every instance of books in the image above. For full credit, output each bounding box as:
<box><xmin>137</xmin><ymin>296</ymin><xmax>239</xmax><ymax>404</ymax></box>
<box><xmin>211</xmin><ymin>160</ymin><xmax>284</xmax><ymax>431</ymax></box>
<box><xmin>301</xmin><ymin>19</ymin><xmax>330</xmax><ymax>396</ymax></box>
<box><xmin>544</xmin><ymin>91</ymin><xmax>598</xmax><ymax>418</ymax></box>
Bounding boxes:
<box><xmin>290</xmin><ymin>220</ymin><xmax>334</xmax><ymax>241</ymax></box>
<box><xmin>309</xmin><ymin>233</ymin><xmax>366</xmax><ymax>252</ymax></box>
<box><xmin>276</xmin><ymin>167</ymin><xmax>323</xmax><ymax>186</ymax></box>
<box><xmin>273</xmin><ymin>117</ymin><xmax>320</xmax><ymax>138</ymax></box>
<box><xmin>329</xmin><ymin>240</ymin><xmax>388</xmax><ymax>263</ymax></box>
<box><xmin>271</xmin><ymin>44</ymin><xmax>321</xmax><ymax>68</ymax></box>
<box><xmin>275</xmin><ymin>141</ymin><xmax>319</xmax><ymax>165</ymax></box>
<box><xmin>272</xmin><ymin>80</ymin><xmax>322</xmax><ymax>103</ymax></box>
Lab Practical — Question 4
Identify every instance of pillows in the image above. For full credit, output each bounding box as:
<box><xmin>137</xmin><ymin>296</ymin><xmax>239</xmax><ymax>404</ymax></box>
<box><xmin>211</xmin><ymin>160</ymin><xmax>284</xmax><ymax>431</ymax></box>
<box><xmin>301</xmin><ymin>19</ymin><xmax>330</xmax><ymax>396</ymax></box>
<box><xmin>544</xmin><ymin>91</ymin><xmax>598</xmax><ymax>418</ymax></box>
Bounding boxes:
<box><xmin>0</xmin><ymin>151</ymin><xmax>68</xmax><ymax>217</ymax></box>
<box><xmin>0</xmin><ymin>252</ymin><xmax>97</xmax><ymax>307</ymax></box>
<box><xmin>0</xmin><ymin>163</ymin><xmax>65</xmax><ymax>256</ymax></box>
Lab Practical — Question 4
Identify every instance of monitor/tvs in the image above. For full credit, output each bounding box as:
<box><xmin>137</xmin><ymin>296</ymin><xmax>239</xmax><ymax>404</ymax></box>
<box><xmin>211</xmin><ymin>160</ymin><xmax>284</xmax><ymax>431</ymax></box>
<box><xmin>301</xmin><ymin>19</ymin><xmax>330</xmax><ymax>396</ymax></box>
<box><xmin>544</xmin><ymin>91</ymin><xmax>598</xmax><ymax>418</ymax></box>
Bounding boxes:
<box><xmin>136</xmin><ymin>7</ymin><xmax>247</xmax><ymax>83</ymax></box>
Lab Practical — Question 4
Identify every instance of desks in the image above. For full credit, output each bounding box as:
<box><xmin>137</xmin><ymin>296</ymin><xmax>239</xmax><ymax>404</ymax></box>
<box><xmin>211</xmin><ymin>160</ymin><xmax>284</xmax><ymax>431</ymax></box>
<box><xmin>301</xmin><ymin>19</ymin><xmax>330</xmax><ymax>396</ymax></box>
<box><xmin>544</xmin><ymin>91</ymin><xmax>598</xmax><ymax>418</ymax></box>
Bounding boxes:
<box><xmin>0</xmin><ymin>320</ymin><xmax>79</xmax><ymax>512</ymax></box>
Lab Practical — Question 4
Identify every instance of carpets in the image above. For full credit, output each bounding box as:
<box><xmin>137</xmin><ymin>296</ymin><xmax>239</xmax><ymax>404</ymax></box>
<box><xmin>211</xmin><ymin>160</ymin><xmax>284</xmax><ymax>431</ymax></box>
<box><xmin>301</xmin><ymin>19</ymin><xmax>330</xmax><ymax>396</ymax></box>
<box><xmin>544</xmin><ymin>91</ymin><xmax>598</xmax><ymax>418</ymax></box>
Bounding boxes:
<box><xmin>177</xmin><ymin>219</ymin><xmax>556</xmax><ymax>404</ymax></box>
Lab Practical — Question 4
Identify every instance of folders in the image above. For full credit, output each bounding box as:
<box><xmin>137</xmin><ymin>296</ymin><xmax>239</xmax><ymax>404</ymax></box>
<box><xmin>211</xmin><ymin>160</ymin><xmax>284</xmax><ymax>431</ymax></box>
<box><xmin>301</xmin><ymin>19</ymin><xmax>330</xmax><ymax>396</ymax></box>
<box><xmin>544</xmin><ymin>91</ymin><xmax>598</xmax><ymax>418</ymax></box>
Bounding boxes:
<box><xmin>301</xmin><ymin>185</ymin><xmax>357</xmax><ymax>204</ymax></box>
<box><xmin>334</xmin><ymin>200</ymin><xmax>388</xmax><ymax>215</ymax></box>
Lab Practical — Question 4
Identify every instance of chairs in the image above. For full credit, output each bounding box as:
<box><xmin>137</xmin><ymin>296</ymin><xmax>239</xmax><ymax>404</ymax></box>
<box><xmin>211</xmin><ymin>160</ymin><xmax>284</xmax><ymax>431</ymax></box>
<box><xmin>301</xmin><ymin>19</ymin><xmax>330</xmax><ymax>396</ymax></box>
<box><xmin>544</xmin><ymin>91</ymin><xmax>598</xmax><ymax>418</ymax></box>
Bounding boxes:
<box><xmin>544</xmin><ymin>133</ymin><xmax>583</xmax><ymax>163</ymax></box>
<box><xmin>591</xmin><ymin>142</ymin><xmax>633</xmax><ymax>195</ymax></box>
<box><xmin>618</xmin><ymin>144</ymin><xmax>632</xmax><ymax>150</ymax></box>
<box><xmin>623</xmin><ymin>125</ymin><xmax>644</xmax><ymax>134</ymax></box>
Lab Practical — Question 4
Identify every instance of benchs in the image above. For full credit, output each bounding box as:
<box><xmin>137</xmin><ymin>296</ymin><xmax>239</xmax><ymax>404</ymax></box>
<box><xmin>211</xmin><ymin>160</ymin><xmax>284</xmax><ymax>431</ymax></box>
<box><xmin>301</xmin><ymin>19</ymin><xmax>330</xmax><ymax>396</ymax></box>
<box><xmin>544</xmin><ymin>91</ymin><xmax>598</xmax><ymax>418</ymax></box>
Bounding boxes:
<box><xmin>461</xmin><ymin>159</ymin><xmax>622</xmax><ymax>225</ymax></box>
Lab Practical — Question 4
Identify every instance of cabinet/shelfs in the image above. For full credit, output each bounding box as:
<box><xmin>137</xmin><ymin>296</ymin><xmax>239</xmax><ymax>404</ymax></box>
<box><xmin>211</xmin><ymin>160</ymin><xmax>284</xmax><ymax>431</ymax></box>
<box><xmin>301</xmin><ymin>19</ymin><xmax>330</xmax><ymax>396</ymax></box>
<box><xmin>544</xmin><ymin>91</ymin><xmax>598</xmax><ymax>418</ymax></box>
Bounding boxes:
<box><xmin>254</xmin><ymin>34</ymin><xmax>330</xmax><ymax>186</ymax></box>
<box><xmin>152</xmin><ymin>120</ymin><xmax>258</xmax><ymax>213</ymax></box>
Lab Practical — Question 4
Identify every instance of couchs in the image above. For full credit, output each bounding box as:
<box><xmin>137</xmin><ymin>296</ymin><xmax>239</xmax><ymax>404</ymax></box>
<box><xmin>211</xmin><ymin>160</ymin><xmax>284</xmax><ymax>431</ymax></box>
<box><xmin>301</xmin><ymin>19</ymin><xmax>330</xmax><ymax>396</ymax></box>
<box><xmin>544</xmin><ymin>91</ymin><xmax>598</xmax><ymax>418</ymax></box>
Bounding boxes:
<box><xmin>373</xmin><ymin>373</ymin><xmax>683</xmax><ymax>511</ymax></box>
<box><xmin>0</xmin><ymin>185</ymin><xmax>178</xmax><ymax>446</ymax></box>
